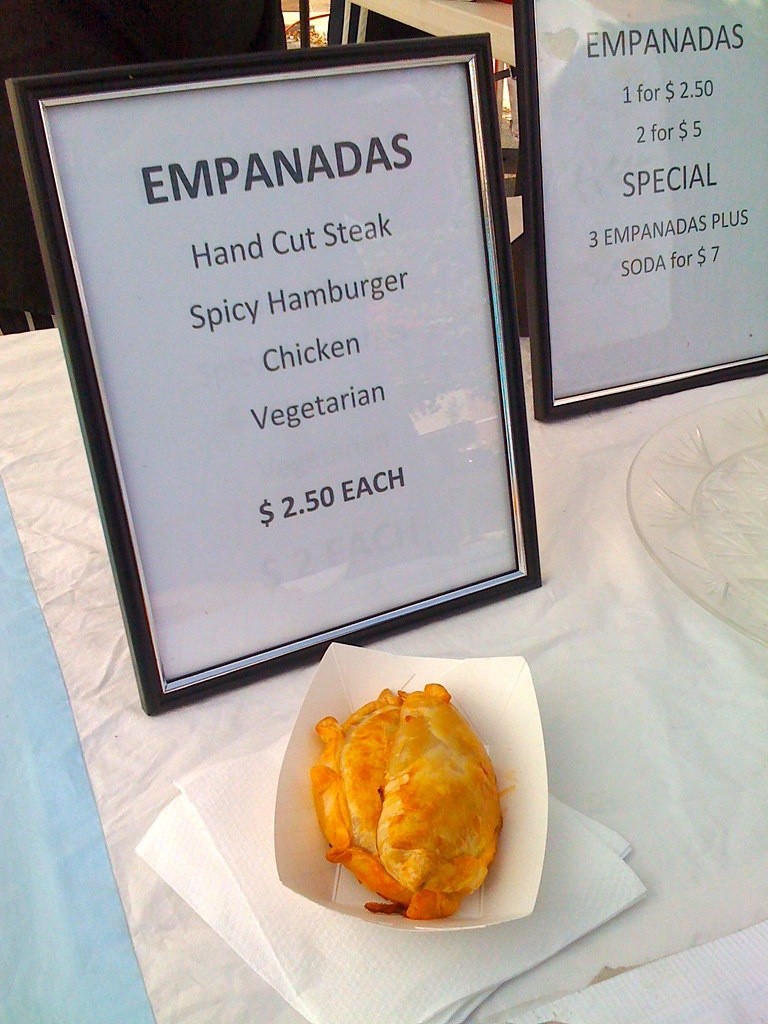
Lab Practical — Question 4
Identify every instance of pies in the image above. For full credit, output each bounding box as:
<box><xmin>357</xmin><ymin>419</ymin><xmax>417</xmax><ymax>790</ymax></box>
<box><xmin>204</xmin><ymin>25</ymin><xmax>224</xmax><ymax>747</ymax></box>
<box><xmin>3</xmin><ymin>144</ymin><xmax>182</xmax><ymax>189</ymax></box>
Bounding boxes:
<box><xmin>310</xmin><ymin>684</ymin><xmax>501</xmax><ymax>920</ymax></box>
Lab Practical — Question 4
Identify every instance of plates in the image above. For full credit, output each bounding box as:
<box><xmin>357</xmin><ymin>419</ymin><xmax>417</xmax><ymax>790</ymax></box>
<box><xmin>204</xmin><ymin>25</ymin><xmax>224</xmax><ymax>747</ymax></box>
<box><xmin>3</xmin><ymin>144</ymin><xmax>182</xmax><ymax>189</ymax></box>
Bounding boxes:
<box><xmin>627</xmin><ymin>391</ymin><xmax>768</xmax><ymax>649</ymax></box>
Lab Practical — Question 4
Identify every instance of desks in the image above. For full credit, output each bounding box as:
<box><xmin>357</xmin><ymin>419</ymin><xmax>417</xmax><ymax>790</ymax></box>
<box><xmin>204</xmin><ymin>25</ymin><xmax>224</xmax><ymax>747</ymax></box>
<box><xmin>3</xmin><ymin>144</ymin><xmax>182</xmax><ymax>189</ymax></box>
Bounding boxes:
<box><xmin>0</xmin><ymin>322</ymin><xmax>768</xmax><ymax>1024</ymax></box>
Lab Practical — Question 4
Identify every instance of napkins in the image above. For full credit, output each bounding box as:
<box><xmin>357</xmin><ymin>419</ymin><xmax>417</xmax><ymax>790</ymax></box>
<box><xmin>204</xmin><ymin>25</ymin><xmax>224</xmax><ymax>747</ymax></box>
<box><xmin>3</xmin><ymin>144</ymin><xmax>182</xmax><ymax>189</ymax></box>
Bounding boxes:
<box><xmin>513</xmin><ymin>916</ymin><xmax>768</xmax><ymax>1024</ymax></box>
<box><xmin>133</xmin><ymin>732</ymin><xmax>648</xmax><ymax>1024</ymax></box>
